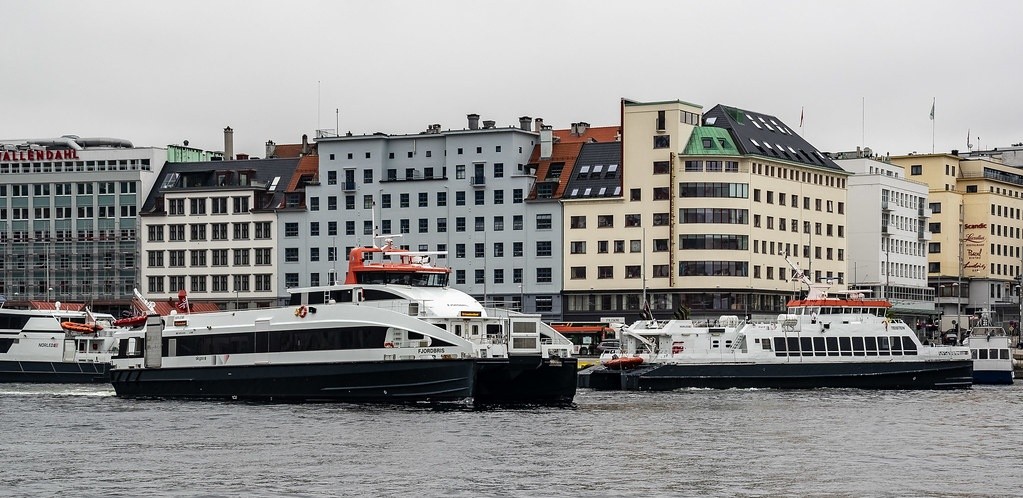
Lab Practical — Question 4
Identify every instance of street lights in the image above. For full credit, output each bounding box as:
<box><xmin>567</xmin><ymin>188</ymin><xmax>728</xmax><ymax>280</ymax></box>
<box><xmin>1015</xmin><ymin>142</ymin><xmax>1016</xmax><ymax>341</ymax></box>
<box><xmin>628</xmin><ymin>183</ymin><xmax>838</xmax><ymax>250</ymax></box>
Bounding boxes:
<box><xmin>937</xmin><ymin>273</ymin><xmax>945</xmax><ymax>344</ymax></box>
<box><xmin>1015</xmin><ymin>279</ymin><xmax>1021</xmax><ymax>343</ymax></box>
<box><xmin>952</xmin><ymin>282</ymin><xmax>963</xmax><ymax>346</ymax></box>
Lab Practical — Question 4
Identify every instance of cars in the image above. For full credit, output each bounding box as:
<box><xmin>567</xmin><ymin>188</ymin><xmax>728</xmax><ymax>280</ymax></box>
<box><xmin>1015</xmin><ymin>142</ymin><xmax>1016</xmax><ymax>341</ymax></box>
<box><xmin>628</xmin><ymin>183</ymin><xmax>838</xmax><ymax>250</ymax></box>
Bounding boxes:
<box><xmin>942</xmin><ymin>333</ymin><xmax>957</xmax><ymax>346</ymax></box>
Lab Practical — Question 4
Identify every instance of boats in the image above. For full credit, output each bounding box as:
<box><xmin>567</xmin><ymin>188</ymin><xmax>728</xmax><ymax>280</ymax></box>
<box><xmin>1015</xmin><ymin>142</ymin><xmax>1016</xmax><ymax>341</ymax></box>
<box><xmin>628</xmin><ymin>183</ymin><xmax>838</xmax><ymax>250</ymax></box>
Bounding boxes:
<box><xmin>62</xmin><ymin>323</ymin><xmax>104</xmax><ymax>338</ymax></box>
<box><xmin>602</xmin><ymin>355</ymin><xmax>650</xmax><ymax>369</ymax></box>
<box><xmin>108</xmin><ymin>200</ymin><xmax>583</xmax><ymax>411</ymax></box>
<box><xmin>0</xmin><ymin>301</ymin><xmax>128</xmax><ymax>384</ymax></box>
<box><xmin>588</xmin><ymin>249</ymin><xmax>976</xmax><ymax>392</ymax></box>
<box><xmin>965</xmin><ymin>326</ymin><xmax>1018</xmax><ymax>385</ymax></box>
<box><xmin>113</xmin><ymin>315</ymin><xmax>147</xmax><ymax>329</ymax></box>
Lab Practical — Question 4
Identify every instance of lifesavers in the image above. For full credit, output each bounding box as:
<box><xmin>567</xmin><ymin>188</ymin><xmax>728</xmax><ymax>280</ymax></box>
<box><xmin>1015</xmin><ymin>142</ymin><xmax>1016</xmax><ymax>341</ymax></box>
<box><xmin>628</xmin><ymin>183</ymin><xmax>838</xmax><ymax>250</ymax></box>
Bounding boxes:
<box><xmin>299</xmin><ymin>305</ymin><xmax>307</xmax><ymax>317</ymax></box>
<box><xmin>385</xmin><ymin>343</ymin><xmax>394</xmax><ymax>348</ymax></box>
